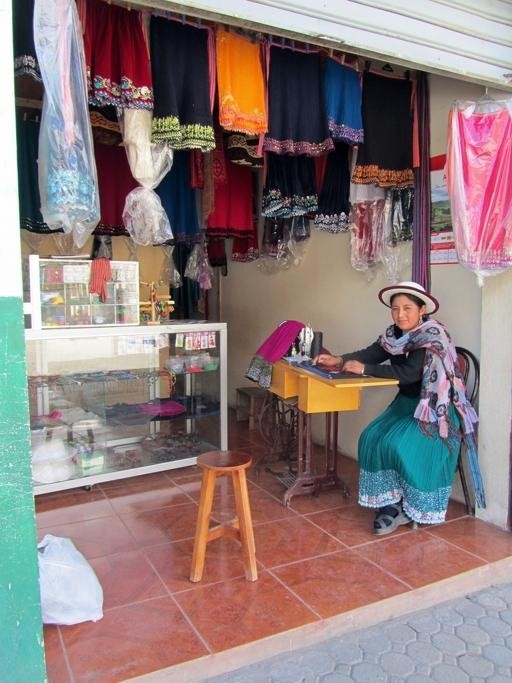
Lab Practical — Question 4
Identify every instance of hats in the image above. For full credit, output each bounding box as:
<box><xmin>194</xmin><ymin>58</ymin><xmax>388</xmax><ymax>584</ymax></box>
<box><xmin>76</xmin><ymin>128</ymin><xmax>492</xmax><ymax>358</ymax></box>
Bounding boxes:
<box><xmin>377</xmin><ymin>282</ymin><xmax>439</xmax><ymax>315</ymax></box>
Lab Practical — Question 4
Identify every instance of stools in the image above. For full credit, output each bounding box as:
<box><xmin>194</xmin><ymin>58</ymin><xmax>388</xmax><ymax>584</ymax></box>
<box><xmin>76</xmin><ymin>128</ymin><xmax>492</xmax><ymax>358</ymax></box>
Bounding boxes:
<box><xmin>187</xmin><ymin>450</ymin><xmax>258</xmax><ymax>585</ymax></box>
<box><xmin>234</xmin><ymin>386</ymin><xmax>277</xmax><ymax>435</ymax></box>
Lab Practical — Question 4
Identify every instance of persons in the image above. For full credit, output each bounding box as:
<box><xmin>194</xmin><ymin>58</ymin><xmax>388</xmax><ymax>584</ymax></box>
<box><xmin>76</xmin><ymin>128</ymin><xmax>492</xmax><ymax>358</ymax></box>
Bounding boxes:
<box><xmin>312</xmin><ymin>281</ymin><xmax>479</xmax><ymax>536</ymax></box>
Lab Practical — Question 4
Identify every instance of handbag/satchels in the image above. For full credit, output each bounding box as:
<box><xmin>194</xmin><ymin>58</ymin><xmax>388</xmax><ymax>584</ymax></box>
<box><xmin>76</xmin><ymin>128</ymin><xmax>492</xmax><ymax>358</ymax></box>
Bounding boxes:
<box><xmin>37</xmin><ymin>534</ymin><xmax>103</xmax><ymax>625</ymax></box>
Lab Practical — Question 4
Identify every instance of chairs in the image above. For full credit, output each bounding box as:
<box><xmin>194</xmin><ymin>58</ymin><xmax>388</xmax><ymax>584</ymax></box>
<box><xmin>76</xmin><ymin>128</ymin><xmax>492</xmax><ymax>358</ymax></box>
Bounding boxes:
<box><xmin>448</xmin><ymin>343</ymin><xmax>482</xmax><ymax>515</ymax></box>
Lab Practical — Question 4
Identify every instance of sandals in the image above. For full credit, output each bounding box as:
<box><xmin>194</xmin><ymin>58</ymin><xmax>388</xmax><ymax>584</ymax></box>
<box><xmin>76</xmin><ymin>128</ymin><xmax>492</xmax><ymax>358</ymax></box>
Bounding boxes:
<box><xmin>373</xmin><ymin>503</ymin><xmax>413</xmax><ymax>536</ymax></box>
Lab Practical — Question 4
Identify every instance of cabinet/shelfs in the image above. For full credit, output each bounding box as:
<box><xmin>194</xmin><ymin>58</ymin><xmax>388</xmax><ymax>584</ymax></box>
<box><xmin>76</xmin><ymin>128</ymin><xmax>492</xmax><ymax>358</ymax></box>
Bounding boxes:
<box><xmin>28</xmin><ymin>251</ymin><xmax>142</xmax><ymax>330</ymax></box>
<box><xmin>23</xmin><ymin>317</ymin><xmax>228</xmax><ymax>497</ymax></box>
